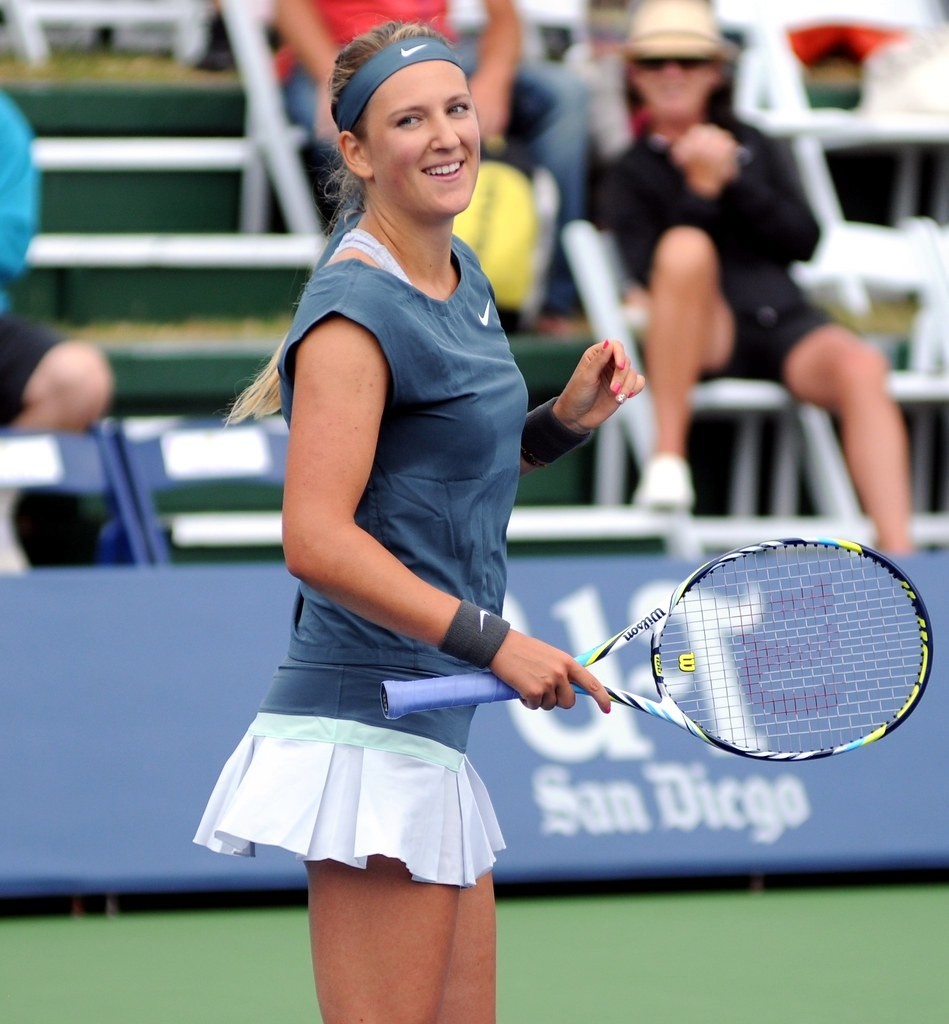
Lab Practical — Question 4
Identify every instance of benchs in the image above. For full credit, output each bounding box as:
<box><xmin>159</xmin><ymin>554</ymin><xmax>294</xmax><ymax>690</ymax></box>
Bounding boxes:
<box><xmin>0</xmin><ymin>0</ymin><xmax>949</xmax><ymax>557</ymax></box>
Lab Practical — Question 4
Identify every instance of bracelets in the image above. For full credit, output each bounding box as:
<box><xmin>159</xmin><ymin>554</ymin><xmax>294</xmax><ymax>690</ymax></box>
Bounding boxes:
<box><xmin>437</xmin><ymin>599</ymin><xmax>511</xmax><ymax>670</ymax></box>
<box><xmin>521</xmin><ymin>448</ymin><xmax>546</xmax><ymax>468</ymax></box>
<box><xmin>521</xmin><ymin>397</ymin><xmax>590</xmax><ymax>463</ymax></box>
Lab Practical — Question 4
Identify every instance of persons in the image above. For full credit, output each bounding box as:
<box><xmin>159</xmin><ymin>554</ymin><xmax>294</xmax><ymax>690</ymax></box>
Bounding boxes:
<box><xmin>229</xmin><ymin>0</ymin><xmax>932</xmax><ymax>559</ymax></box>
<box><xmin>195</xmin><ymin>23</ymin><xmax>646</xmax><ymax>1024</ymax></box>
<box><xmin>0</xmin><ymin>91</ymin><xmax>115</xmax><ymax>572</ymax></box>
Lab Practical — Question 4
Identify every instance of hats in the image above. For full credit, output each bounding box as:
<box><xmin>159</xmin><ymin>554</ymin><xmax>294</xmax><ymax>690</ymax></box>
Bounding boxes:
<box><xmin>615</xmin><ymin>0</ymin><xmax>739</xmax><ymax>63</ymax></box>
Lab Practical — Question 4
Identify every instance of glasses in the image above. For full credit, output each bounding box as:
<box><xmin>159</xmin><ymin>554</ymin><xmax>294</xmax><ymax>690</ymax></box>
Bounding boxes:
<box><xmin>635</xmin><ymin>57</ymin><xmax>718</xmax><ymax>71</ymax></box>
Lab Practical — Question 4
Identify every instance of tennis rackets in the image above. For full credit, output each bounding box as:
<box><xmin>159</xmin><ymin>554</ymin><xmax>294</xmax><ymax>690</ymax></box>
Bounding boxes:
<box><xmin>380</xmin><ymin>537</ymin><xmax>935</xmax><ymax>760</ymax></box>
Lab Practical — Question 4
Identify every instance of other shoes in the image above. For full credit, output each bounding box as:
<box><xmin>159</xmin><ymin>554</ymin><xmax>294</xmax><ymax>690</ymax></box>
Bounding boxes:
<box><xmin>634</xmin><ymin>456</ymin><xmax>696</xmax><ymax>509</ymax></box>
